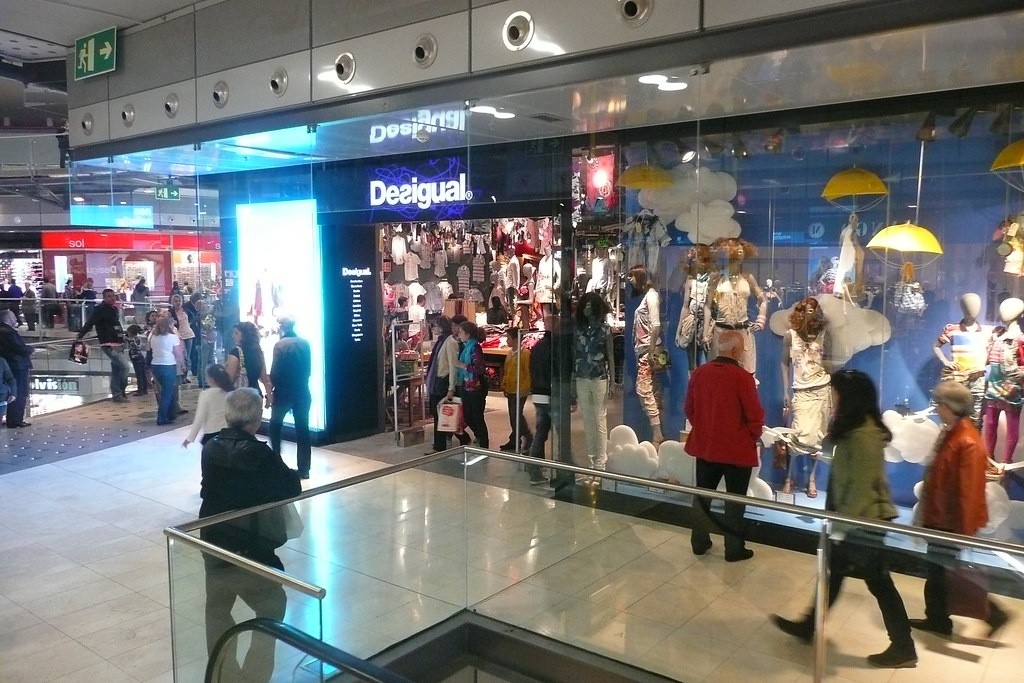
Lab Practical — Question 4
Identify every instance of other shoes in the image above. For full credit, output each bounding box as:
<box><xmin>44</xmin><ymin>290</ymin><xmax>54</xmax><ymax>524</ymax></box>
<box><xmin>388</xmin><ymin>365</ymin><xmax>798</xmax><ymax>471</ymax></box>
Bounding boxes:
<box><xmin>499</xmin><ymin>443</ymin><xmax>521</xmax><ymax>449</ymax></box>
<box><xmin>133</xmin><ymin>389</ymin><xmax>147</xmax><ymax>396</ymax></box>
<box><xmin>424</xmin><ymin>449</ymin><xmax>437</xmax><ymax>455</ymax></box>
<box><xmin>433</xmin><ymin>439</ymin><xmax>452</xmax><ymax>446</ymax></box>
<box><xmin>157</xmin><ymin>409</ymin><xmax>188</xmax><ymax>425</ymax></box>
<box><xmin>7</xmin><ymin>420</ymin><xmax>32</xmax><ymax>428</ymax></box>
<box><xmin>296</xmin><ymin>469</ymin><xmax>309</xmax><ymax>479</ymax></box>
<box><xmin>114</xmin><ymin>395</ymin><xmax>130</xmax><ymax>403</ymax></box>
<box><xmin>524</xmin><ymin>435</ymin><xmax>533</xmax><ymax>451</ymax></box>
<box><xmin>909</xmin><ymin>618</ymin><xmax>952</xmax><ymax>636</ymax></box>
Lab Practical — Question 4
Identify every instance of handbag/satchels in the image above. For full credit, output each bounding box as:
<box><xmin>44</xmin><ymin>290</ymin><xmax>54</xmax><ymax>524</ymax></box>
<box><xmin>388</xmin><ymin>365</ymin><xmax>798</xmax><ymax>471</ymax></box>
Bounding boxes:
<box><xmin>465</xmin><ymin>378</ymin><xmax>481</xmax><ymax>391</ymax></box>
<box><xmin>678</xmin><ymin>313</ymin><xmax>698</xmax><ymax>349</ymax></box>
<box><xmin>229</xmin><ymin>345</ymin><xmax>248</xmax><ymax>389</ymax></box>
<box><xmin>650</xmin><ymin>351</ymin><xmax>669</xmax><ymax>373</ymax></box>
<box><xmin>774</xmin><ymin>440</ymin><xmax>788</xmax><ymax>471</ymax></box>
<box><xmin>437</xmin><ymin>397</ymin><xmax>464</xmax><ymax>436</ymax></box>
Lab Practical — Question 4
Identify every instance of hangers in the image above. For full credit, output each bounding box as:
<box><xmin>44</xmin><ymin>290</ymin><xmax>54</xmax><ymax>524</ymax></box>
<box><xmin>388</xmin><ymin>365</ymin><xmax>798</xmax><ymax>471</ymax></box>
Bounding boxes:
<box><xmin>637</xmin><ymin>201</ymin><xmax>655</xmax><ymax>217</ymax></box>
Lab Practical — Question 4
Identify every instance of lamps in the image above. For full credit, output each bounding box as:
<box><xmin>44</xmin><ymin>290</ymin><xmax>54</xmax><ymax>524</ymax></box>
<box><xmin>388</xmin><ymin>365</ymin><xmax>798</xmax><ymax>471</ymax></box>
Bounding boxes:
<box><xmin>947</xmin><ymin>102</ymin><xmax>984</xmax><ymax>139</ymax></box>
<box><xmin>701</xmin><ymin>137</ymin><xmax>725</xmax><ymax>159</ymax></box>
<box><xmin>989</xmin><ymin>103</ymin><xmax>1013</xmax><ymax>136</ymax></box>
<box><xmin>672</xmin><ymin>139</ymin><xmax>696</xmax><ymax>163</ymax></box>
<box><xmin>731</xmin><ymin>135</ymin><xmax>752</xmax><ymax>161</ymax></box>
<box><xmin>916</xmin><ymin>111</ymin><xmax>936</xmax><ymax>142</ymax></box>
<box><xmin>765</xmin><ymin>128</ymin><xmax>784</xmax><ymax>153</ymax></box>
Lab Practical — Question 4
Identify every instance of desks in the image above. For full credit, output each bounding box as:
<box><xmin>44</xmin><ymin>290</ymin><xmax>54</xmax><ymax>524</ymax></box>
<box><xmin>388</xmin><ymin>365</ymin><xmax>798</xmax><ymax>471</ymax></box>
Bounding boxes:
<box><xmin>386</xmin><ymin>373</ymin><xmax>427</xmax><ymax>428</ymax></box>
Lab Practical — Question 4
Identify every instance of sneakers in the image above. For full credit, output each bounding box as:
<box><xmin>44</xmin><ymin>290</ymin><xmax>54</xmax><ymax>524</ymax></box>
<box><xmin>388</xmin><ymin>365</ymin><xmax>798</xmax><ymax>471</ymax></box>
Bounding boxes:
<box><xmin>530</xmin><ymin>474</ymin><xmax>550</xmax><ymax>484</ymax></box>
<box><xmin>866</xmin><ymin>638</ymin><xmax>918</xmax><ymax>668</ymax></box>
<box><xmin>775</xmin><ymin>616</ymin><xmax>814</xmax><ymax>643</ymax></box>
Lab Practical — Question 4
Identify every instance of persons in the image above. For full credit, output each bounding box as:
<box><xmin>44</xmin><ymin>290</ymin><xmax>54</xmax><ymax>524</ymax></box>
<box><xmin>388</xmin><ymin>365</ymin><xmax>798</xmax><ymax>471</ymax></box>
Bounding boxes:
<box><xmin>933</xmin><ymin>294</ymin><xmax>995</xmax><ymax>436</ymax></box>
<box><xmin>771</xmin><ymin>371</ymin><xmax>917</xmax><ymax>669</ymax></box>
<box><xmin>819</xmin><ymin>256</ymin><xmax>856</xmax><ymax>315</ymax></box>
<box><xmin>390</xmin><ymin>293</ymin><xmax>426</xmax><ymax>403</ymax></box>
<box><xmin>629</xmin><ymin>265</ymin><xmax>671</xmax><ymax>442</ymax></box>
<box><xmin>986</xmin><ymin>299</ymin><xmax>1024</xmax><ymax>469</ymax></box>
<box><xmin>782</xmin><ymin>299</ymin><xmax>836</xmax><ymax>499</ymax></box>
<box><xmin>498</xmin><ymin>327</ymin><xmax>534</xmax><ymax>454</ymax></box>
<box><xmin>424</xmin><ymin>315</ymin><xmax>489</xmax><ymax>453</ymax></box>
<box><xmin>487</xmin><ymin>297</ymin><xmax>508</xmax><ymax>325</ymax></box>
<box><xmin>674</xmin><ymin>244</ymin><xmax>719</xmax><ymax>383</ymax></box>
<box><xmin>908</xmin><ymin>381</ymin><xmax>1008</xmax><ymax>637</ymax></box>
<box><xmin>684</xmin><ymin>331</ymin><xmax>766</xmax><ymax>561</ymax></box>
<box><xmin>591</xmin><ymin>243</ymin><xmax>613</xmax><ymax>291</ymax></box>
<box><xmin>528</xmin><ymin>314</ymin><xmax>578</xmax><ymax>484</ymax></box>
<box><xmin>570</xmin><ymin>293</ymin><xmax>617</xmax><ymax>488</ymax></box>
<box><xmin>180</xmin><ymin>363</ymin><xmax>236</xmax><ymax>449</ymax></box>
<box><xmin>225</xmin><ymin>322</ymin><xmax>274</xmax><ymax>405</ymax></box>
<box><xmin>0</xmin><ymin>275</ymin><xmax>217</xmax><ymax>430</ymax></box>
<box><xmin>269</xmin><ymin>317</ymin><xmax>312</xmax><ymax>479</ymax></box>
<box><xmin>762</xmin><ymin>279</ymin><xmax>782</xmax><ymax>315</ymax></box>
<box><xmin>198</xmin><ymin>389</ymin><xmax>305</xmax><ymax>683</ymax></box>
<box><xmin>703</xmin><ymin>240</ymin><xmax>767</xmax><ymax>392</ymax></box>
<box><xmin>488</xmin><ymin>245</ymin><xmax>561</xmax><ymax>329</ymax></box>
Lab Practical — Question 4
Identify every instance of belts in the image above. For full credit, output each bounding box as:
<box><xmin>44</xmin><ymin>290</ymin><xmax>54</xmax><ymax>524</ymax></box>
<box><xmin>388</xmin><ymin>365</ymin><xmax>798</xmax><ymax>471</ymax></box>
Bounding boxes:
<box><xmin>715</xmin><ymin>319</ymin><xmax>750</xmax><ymax>331</ymax></box>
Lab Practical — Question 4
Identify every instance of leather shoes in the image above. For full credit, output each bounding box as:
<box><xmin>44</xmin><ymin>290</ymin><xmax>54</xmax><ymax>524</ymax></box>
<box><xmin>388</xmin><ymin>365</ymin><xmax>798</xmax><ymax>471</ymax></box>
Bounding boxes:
<box><xmin>725</xmin><ymin>548</ymin><xmax>753</xmax><ymax>562</ymax></box>
<box><xmin>693</xmin><ymin>541</ymin><xmax>712</xmax><ymax>555</ymax></box>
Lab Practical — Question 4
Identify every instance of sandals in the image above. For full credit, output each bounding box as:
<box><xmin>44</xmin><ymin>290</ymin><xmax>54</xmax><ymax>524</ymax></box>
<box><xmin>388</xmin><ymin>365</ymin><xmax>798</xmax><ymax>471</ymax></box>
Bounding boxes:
<box><xmin>782</xmin><ymin>479</ymin><xmax>794</xmax><ymax>494</ymax></box>
<box><xmin>807</xmin><ymin>480</ymin><xmax>817</xmax><ymax>497</ymax></box>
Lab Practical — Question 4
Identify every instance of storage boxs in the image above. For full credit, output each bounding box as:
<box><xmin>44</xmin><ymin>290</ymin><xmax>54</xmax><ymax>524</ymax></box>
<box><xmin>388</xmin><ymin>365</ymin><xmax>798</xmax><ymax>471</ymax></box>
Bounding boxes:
<box><xmin>396</xmin><ymin>353</ymin><xmax>418</xmax><ymax>374</ymax></box>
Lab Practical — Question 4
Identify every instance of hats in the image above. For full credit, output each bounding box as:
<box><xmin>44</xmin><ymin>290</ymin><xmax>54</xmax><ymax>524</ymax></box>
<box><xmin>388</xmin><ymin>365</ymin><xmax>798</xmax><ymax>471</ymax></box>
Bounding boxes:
<box><xmin>935</xmin><ymin>381</ymin><xmax>973</xmax><ymax>417</ymax></box>
